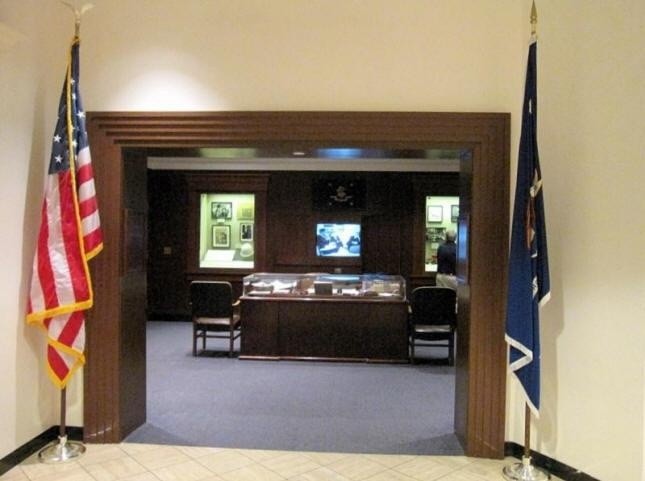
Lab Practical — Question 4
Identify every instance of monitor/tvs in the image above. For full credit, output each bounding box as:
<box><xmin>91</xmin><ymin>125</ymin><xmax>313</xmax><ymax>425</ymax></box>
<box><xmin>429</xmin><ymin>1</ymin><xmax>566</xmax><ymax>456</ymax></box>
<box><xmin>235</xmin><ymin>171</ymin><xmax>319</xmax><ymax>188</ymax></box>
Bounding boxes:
<box><xmin>314</xmin><ymin>219</ymin><xmax>364</xmax><ymax>260</ymax></box>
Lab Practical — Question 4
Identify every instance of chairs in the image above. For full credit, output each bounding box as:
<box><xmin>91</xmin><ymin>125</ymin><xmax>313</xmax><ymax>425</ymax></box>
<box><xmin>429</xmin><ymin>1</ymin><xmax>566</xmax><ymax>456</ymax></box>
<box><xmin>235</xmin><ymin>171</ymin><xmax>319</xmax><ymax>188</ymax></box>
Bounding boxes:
<box><xmin>405</xmin><ymin>284</ymin><xmax>458</xmax><ymax>370</ymax></box>
<box><xmin>188</xmin><ymin>277</ymin><xmax>244</xmax><ymax>359</ymax></box>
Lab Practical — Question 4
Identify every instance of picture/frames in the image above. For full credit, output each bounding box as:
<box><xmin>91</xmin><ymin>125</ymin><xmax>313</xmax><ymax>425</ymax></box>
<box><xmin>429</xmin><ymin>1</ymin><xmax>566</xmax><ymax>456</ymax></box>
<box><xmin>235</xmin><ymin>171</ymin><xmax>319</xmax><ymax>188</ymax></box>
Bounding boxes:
<box><xmin>427</xmin><ymin>204</ymin><xmax>443</xmax><ymax>225</ymax></box>
<box><xmin>210</xmin><ymin>201</ymin><xmax>233</xmax><ymax>220</ymax></box>
<box><xmin>450</xmin><ymin>204</ymin><xmax>460</xmax><ymax>223</ymax></box>
<box><xmin>240</xmin><ymin>221</ymin><xmax>255</xmax><ymax>243</ymax></box>
<box><xmin>210</xmin><ymin>225</ymin><xmax>232</xmax><ymax>250</ymax></box>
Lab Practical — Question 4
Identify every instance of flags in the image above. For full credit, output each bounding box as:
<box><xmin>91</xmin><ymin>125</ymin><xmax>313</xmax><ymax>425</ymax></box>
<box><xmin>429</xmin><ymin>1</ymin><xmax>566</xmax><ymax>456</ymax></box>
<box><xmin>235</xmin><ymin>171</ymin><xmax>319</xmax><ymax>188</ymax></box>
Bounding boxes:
<box><xmin>503</xmin><ymin>35</ymin><xmax>553</xmax><ymax>418</ymax></box>
<box><xmin>25</xmin><ymin>40</ymin><xmax>106</xmax><ymax>390</ymax></box>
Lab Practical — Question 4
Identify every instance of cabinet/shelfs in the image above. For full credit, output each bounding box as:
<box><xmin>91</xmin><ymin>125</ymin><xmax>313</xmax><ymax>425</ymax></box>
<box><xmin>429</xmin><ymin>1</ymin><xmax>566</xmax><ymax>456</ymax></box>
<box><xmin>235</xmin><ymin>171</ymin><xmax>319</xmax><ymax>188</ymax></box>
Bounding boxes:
<box><xmin>236</xmin><ymin>270</ymin><xmax>416</xmax><ymax>367</ymax></box>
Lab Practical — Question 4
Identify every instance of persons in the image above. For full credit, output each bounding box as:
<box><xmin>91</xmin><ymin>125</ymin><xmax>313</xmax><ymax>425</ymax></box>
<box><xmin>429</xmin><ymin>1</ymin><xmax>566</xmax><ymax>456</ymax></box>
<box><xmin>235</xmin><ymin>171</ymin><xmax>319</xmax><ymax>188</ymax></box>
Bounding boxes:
<box><xmin>317</xmin><ymin>228</ymin><xmax>360</xmax><ymax>254</ymax></box>
<box><xmin>437</xmin><ymin>230</ymin><xmax>457</xmax><ymax>315</ymax></box>
